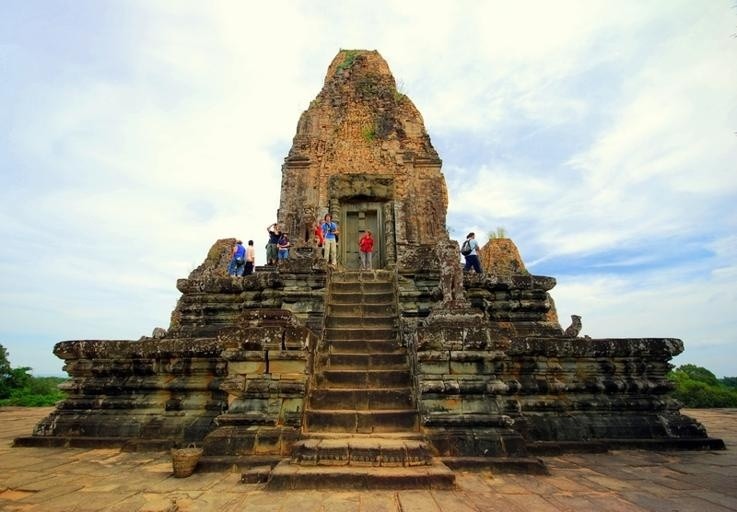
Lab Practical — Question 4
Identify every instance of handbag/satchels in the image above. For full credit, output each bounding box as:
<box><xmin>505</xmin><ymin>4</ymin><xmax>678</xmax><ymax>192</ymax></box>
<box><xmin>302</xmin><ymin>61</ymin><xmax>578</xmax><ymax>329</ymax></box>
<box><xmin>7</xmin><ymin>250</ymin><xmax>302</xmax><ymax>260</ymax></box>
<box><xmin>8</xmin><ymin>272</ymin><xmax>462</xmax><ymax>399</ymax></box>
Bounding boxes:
<box><xmin>335</xmin><ymin>234</ymin><xmax>339</xmax><ymax>243</ymax></box>
<box><xmin>235</xmin><ymin>258</ymin><xmax>244</xmax><ymax>266</ymax></box>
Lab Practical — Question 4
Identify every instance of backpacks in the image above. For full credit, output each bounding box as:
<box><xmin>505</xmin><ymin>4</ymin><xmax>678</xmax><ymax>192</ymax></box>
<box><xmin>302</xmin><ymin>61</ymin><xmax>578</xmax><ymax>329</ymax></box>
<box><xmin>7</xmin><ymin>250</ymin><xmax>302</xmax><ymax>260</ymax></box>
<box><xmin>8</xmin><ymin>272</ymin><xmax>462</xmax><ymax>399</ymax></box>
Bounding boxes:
<box><xmin>461</xmin><ymin>239</ymin><xmax>475</xmax><ymax>256</ymax></box>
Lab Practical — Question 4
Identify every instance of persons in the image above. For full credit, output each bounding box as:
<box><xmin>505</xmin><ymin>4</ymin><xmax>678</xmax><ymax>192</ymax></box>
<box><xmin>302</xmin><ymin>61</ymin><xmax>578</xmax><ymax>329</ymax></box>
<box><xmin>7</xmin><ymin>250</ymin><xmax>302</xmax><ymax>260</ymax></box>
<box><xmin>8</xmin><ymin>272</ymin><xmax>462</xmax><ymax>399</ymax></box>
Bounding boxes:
<box><xmin>241</xmin><ymin>239</ymin><xmax>255</xmax><ymax>277</ymax></box>
<box><xmin>227</xmin><ymin>239</ymin><xmax>246</xmax><ymax>276</ymax></box>
<box><xmin>314</xmin><ymin>219</ymin><xmax>325</xmax><ymax>257</ymax></box>
<box><xmin>321</xmin><ymin>213</ymin><xmax>340</xmax><ymax>269</ymax></box>
<box><xmin>460</xmin><ymin>232</ymin><xmax>482</xmax><ymax>273</ymax></box>
<box><xmin>265</xmin><ymin>222</ymin><xmax>280</xmax><ymax>266</ymax></box>
<box><xmin>358</xmin><ymin>230</ymin><xmax>375</xmax><ymax>272</ymax></box>
<box><xmin>276</xmin><ymin>233</ymin><xmax>290</xmax><ymax>264</ymax></box>
